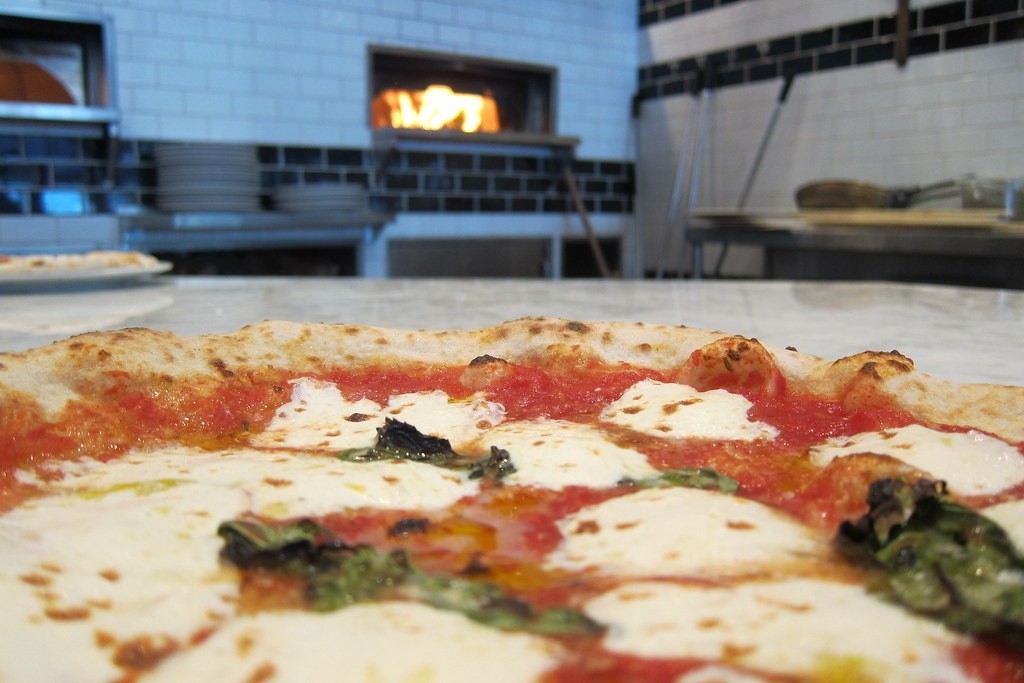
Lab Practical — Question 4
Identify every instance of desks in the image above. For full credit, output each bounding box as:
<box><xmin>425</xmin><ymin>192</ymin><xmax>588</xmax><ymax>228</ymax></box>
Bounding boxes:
<box><xmin>122</xmin><ymin>223</ymin><xmax>376</xmax><ymax>278</ymax></box>
<box><xmin>687</xmin><ymin>225</ymin><xmax>1024</xmax><ymax>280</ymax></box>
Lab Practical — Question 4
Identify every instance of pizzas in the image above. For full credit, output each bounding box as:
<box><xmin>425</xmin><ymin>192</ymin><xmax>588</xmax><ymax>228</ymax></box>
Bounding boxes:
<box><xmin>0</xmin><ymin>316</ymin><xmax>1024</xmax><ymax>683</ymax></box>
<box><xmin>0</xmin><ymin>250</ymin><xmax>159</xmax><ymax>273</ymax></box>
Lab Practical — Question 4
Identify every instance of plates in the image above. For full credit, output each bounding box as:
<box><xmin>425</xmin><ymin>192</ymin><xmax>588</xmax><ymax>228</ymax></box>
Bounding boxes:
<box><xmin>0</xmin><ymin>260</ymin><xmax>172</xmax><ymax>284</ymax></box>
<box><xmin>272</xmin><ymin>181</ymin><xmax>371</xmax><ymax>228</ymax></box>
<box><xmin>151</xmin><ymin>142</ymin><xmax>262</xmax><ymax>213</ymax></box>
